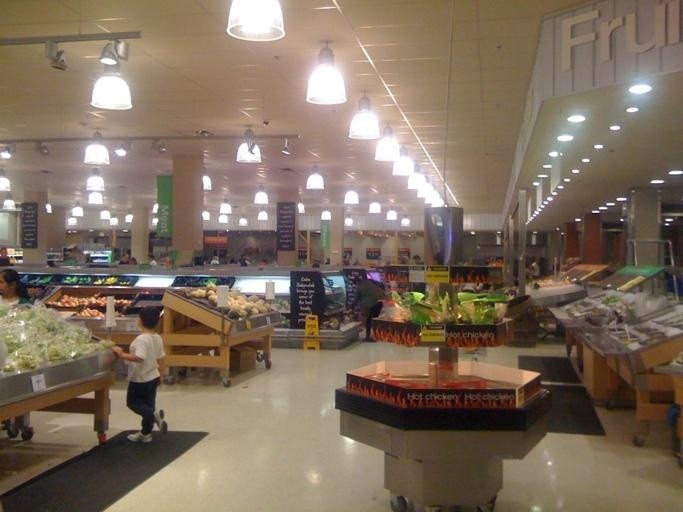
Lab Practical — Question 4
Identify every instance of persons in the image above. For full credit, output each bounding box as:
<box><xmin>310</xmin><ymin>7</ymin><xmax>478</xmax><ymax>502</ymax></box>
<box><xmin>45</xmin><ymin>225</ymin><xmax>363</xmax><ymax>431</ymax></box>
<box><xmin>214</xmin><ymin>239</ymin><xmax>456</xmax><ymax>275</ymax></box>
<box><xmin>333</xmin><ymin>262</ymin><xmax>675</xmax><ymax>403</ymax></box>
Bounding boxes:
<box><xmin>206</xmin><ymin>252</ymin><xmax>425</xmax><ymax>270</ymax></box>
<box><xmin>345</xmin><ymin>277</ymin><xmax>384</xmax><ymax>343</ymax></box>
<box><xmin>529</xmin><ymin>255</ymin><xmax>542</xmax><ymax>277</ymax></box>
<box><xmin>62</xmin><ymin>246</ymin><xmax>157</xmax><ymax>265</ymax></box>
<box><xmin>110</xmin><ymin>305</ymin><xmax>168</xmax><ymax>445</ymax></box>
<box><xmin>0</xmin><ymin>247</ymin><xmax>14</xmax><ymax>266</ymax></box>
<box><xmin>0</xmin><ymin>266</ymin><xmax>32</xmax><ymax>306</ymax></box>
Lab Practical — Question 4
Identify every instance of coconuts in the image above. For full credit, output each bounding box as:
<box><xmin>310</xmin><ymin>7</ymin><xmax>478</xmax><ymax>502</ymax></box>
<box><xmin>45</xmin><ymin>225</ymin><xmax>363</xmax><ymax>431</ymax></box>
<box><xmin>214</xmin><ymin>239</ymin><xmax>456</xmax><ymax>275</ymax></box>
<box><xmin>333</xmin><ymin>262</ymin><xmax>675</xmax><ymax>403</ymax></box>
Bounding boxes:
<box><xmin>191</xmin><ymin>283</ymin><xmax>271</xmax><ymax>318</ymax></box>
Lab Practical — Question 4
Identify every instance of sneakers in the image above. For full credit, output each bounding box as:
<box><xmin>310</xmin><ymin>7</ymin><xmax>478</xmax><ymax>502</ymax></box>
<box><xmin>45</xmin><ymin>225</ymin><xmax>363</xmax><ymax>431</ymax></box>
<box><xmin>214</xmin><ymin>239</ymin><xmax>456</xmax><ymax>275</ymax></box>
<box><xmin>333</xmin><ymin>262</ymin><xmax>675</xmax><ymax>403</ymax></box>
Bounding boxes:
<box><xmin>128</xmin><ymin>432</ymin><xmax>152</xmax><ymax>443</ymax></box>
<box><xmin>153</xmin><ymin>409</ymin><xmax>167</xmax><ymax>433</ymax></box>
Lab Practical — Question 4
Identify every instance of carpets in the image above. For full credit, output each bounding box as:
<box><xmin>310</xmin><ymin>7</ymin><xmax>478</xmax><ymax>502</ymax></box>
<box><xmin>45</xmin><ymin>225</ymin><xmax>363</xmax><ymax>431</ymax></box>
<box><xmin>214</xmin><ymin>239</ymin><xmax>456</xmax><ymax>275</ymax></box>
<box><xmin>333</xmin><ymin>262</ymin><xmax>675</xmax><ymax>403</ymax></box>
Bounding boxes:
<box><xmin>518</xmin><ymin>354</ymin><xmax>604</xmax><ymax>434</ymax></box>
<box><xmin>0</xmin><ymin>430</ymin><xmax>209</xmax><ymax>512</ymax></box>
